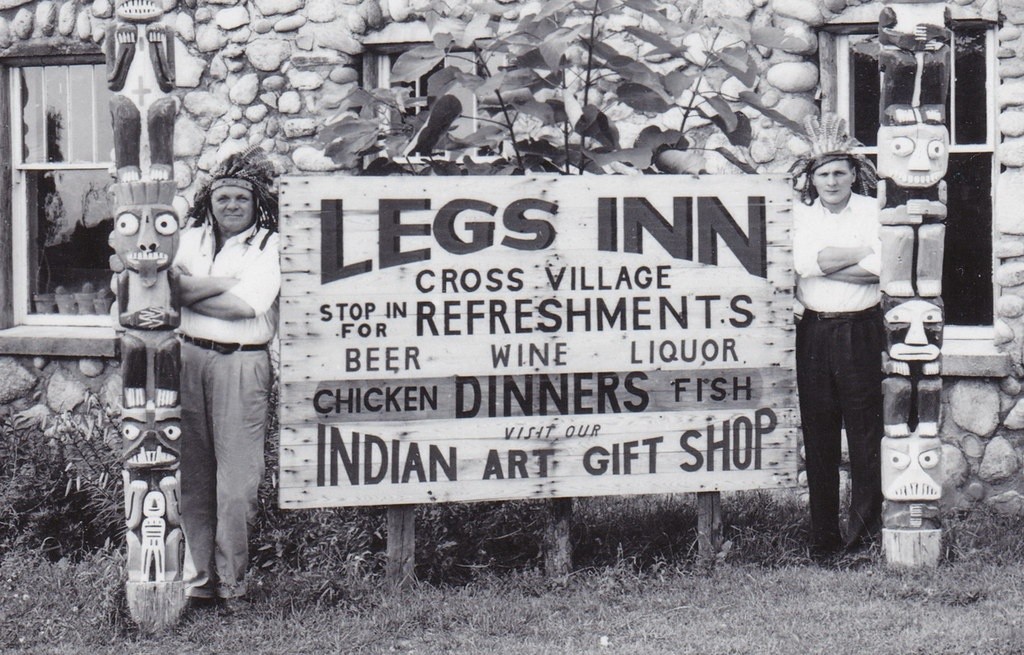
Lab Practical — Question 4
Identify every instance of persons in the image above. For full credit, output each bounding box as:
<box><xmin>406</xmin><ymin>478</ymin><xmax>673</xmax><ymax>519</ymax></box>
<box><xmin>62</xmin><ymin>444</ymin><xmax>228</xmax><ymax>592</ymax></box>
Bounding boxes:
<box><xmin>791</xmin><ymin>115</ymin><xmax>882</xmax><ymax>562</ymax></box>
<box><xmin>171</xmin><ymin>145</ymin><xmax>281</xmax><ymax>616</ymax></box>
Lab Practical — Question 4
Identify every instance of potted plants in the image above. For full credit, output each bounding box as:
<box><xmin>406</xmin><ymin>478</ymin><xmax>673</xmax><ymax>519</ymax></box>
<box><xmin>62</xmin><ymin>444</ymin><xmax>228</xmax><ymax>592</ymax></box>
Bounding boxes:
<box><xmin>93</xmin><ymin>289</ymin><xmax>113</xmax><ymax>315</ymax></box>
<box><xmin>34</xmin><ymin>191</ymin><xmax>64</xmax><ymax>314</ymax></box>
<box><xmin>75</xmin><ymin>283</ymin><xmax>97</xmax><ymax>314</ymax></box>
<box><xmin>55</xmin><ymin>285</ymin><xmax>78</xmax><ymax>316</ymax></box>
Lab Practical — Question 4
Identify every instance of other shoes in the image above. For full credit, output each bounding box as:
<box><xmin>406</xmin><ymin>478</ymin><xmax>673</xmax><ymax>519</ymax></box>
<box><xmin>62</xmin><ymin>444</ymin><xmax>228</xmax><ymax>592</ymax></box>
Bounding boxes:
<box><xmin>218</xmin><ymin>596</ymin><xmax>252</xmax><ymax>617</ymax></box>
<box><xmin>186</xmin><ymin>596</ymin><xmax>217</xmax><ymax>607</ymax></box>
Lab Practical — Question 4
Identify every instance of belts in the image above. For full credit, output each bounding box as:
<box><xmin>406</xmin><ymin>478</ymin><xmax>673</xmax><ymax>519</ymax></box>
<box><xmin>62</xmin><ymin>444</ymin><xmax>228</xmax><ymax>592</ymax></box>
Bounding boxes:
<box><xmin>803</xmin><ymin>304</ymin><xmax>881</xmax><ymax>319</ymax></box>
<box><xmin>179</xmin><ymin>331</ymin><xmax>267</xmax><ymax>355</ymax></box>
